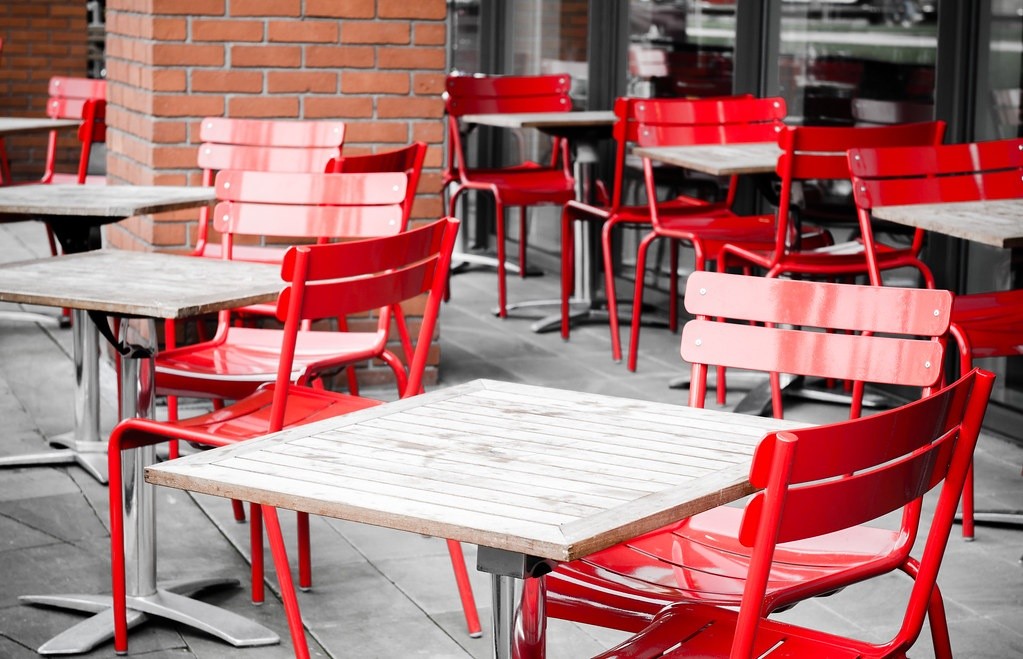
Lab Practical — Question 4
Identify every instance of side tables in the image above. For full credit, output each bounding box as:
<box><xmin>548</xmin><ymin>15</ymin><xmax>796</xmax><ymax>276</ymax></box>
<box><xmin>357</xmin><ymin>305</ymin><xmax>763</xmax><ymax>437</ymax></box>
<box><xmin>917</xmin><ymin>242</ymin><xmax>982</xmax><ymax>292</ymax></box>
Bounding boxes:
<box><xmin>0</xmin><ymin>182</ymin><xmax>217</xmax><ymax>486</ymax></box>
<box><xmin>139</xmin><ymin>378</ymin><xmax>826</xmax><ymax>659</ymax></box>
<box><xmin>0</xmin><ymin>116</ymin><xmax>84</xmax><ymax>185</ymax></box>
<box><xmin>0</xmin><ymin>246</ymin><xmax>292</xmax><ymax>656</ymax></box>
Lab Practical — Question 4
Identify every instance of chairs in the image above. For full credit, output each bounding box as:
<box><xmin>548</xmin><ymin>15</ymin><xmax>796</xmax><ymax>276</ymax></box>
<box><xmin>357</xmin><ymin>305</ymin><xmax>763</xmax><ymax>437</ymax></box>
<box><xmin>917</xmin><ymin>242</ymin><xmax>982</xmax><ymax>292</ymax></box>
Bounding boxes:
<box><xmin>440</xmin><ymin>71</ymin><xmax>1022</xmax><ymax>546</ymax></box>
<box><xmin>513</xmin><ymin>268</ymin><xmax>957</xmax><ymax>659</ymax></box>
<box><xmin>57</xmin><ymin>98</ymin><xmax>107</xmax><ymax>329</ymax></box>
<box><xmin>8</xmin><ymin>76</ymin><xmax>105</xmax><ymax>185</ymax></box>
<box><xmin>106</xmin><ymin>115</ymin><xmax>483</xmax><ymax>658</ymax></box>
<box><xmin>587</xmin><ymin>367</ymin><xmax>1000</xmax><ymax>659</ymax></box>
